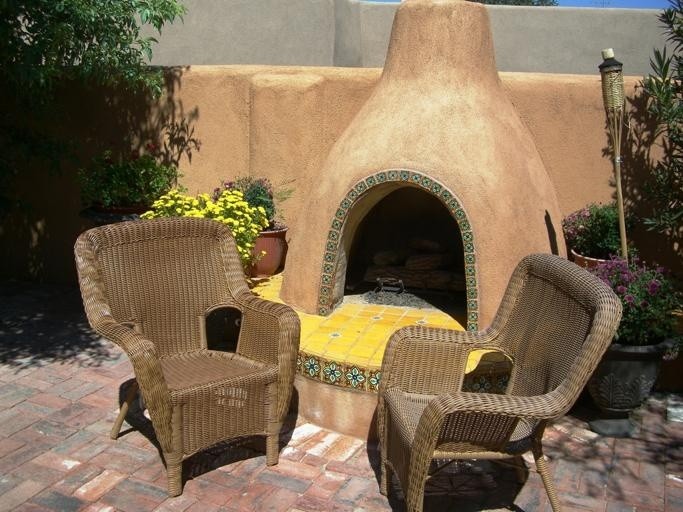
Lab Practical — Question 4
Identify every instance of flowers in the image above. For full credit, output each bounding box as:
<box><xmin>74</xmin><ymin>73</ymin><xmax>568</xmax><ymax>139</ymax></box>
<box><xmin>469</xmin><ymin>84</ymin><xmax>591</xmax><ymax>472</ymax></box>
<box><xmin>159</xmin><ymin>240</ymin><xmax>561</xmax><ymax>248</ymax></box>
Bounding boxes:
<box><xmin>580</xmin><ymin>258</ymin><xmax>682</xmax><ymax>345</ymax></box>
<box><xmin>80</xmin><ymin>135</ymin><xmax>174</xmax><ymax>209</ymax></box>
<box><xmin>210</xmin><ymin>173</ymin><xmax>283</xmax><ymax>228</ymax></box>
<box><xmin>561</xmin><ymin>202</ymin><xmax>635</xmax><ymax>256</ymax></box>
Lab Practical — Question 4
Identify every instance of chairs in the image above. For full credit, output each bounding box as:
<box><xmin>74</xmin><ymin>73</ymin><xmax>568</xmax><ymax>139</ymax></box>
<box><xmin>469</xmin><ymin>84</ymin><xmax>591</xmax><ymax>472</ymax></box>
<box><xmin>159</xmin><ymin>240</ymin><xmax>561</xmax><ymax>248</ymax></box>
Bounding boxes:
<box><xmin>73</xmin><ymin>218</ymin><xmax>301</xmax><ymax>498</ymax></box>
<box><xmin>376</xmin><ymin>251</ymin><xmax>623</xmax><ymax>509</ymax></box>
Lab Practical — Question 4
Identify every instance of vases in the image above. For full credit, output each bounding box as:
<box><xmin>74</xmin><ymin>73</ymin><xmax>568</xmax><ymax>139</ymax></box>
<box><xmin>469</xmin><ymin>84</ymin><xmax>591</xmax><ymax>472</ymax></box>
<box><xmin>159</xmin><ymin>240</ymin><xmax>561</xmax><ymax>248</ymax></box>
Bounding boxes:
<box><xmin>240</xmin><ymin>222</ymin><xmax>288</xmax><ymax>279</ymax></box>
<box><xmin>586</xmin><ymin>339</ymin><xmax>662</xmax><ymax>417</ymax></box>
<box><xmin>569</xmin><ymin>239</ymin><xmax>605</xmax><ymax>266</ymax></box>
<box><xmin>93</xmin><ymin>198</ymin><xmax>149</xmax><ymax>223</ymax></box>
